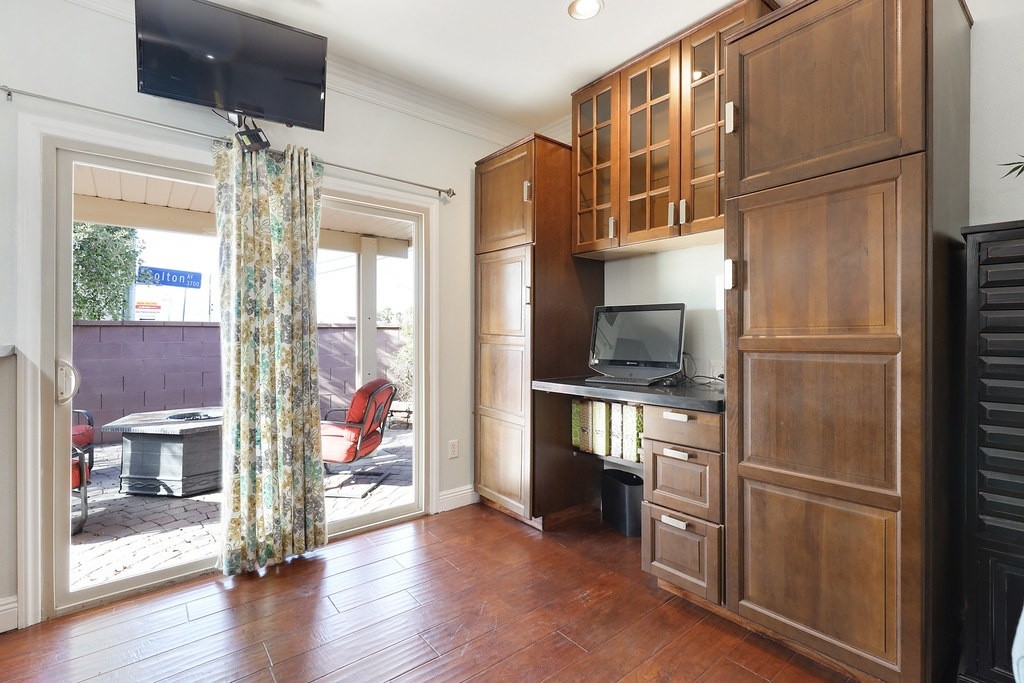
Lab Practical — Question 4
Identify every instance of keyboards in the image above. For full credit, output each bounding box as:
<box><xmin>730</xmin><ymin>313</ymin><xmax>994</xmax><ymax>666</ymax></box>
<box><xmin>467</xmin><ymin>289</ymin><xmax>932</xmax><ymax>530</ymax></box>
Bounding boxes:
<box><xmin>584</xmin><ymin>376</ymin><xmax>665</xmax><ymax>386</ymax></box>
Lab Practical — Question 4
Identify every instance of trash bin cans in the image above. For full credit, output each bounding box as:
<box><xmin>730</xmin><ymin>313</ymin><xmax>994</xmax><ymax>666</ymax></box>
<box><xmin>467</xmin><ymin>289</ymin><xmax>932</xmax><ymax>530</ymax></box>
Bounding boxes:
<box><xmin>602</xmin><ymin>469</ymin><xmax>644</xmax><ymax>536</ymax></box>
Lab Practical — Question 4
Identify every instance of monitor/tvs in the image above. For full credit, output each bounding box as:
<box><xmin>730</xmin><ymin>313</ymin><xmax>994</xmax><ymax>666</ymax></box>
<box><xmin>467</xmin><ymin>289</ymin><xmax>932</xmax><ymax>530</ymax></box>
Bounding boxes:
<box><xmin>587</xmin><ymin>303</ymin><xmax>685</xmax><ymax>380</ymax></box>
<box><xmin>135</xmin><ymin>0</ymin><xmax>328</xmax><ymax>132</ymax></box>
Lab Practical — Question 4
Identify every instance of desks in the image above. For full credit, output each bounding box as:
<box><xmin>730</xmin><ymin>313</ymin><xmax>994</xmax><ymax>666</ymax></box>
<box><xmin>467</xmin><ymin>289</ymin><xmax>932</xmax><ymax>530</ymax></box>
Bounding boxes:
<box><xmin>101</xmin><ymin>407</ymin><xmax>228</xmax><ymax>497</ymax></box>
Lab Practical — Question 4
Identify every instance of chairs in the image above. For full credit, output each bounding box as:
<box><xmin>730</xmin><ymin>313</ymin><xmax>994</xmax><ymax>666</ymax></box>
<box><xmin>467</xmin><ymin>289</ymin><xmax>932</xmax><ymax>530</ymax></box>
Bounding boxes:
<box><xmin>71</xmin><ymin>407</ymin><xmax>94</xmax><ymax>535</ymax></box>
<box><xmin>317</xmin><ymin>380</ymin><xmax>398</xmax><ymax>499</ymax></box>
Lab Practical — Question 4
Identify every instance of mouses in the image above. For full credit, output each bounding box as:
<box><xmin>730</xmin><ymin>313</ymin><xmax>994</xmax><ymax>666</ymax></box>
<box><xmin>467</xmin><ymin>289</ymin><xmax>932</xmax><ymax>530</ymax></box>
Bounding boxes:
<box><xmin>663</xmin><ymin>377</ymin><xmax>678</xmax><ymax>386</ymax></box>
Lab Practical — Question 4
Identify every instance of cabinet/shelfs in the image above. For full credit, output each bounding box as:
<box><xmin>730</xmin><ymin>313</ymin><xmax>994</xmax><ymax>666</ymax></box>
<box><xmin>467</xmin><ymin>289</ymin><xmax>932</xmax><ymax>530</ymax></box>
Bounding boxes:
<box><xmin>469</xmin><ymin>0</ymin><xmax>979</xmax><ymax>680</ymax></box>
